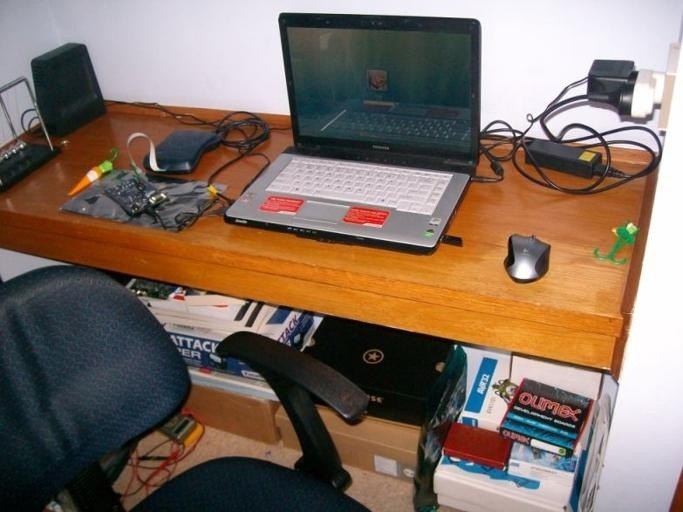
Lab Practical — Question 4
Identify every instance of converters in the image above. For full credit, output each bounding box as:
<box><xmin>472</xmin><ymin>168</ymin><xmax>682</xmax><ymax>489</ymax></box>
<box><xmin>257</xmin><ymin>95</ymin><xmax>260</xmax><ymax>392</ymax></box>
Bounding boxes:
<box><xmin>524</xmin><ymin>139</ymin><xmax>602</xmax><ymax>178</ymax></box>
<box><xmin>586</xmin><ymin>60</ymin><xmax>635</xmax><ymax>102</ymax></box>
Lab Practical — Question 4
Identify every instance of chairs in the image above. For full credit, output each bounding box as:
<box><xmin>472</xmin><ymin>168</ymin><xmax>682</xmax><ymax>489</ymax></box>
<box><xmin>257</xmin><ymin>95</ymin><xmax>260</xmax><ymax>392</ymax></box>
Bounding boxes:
<box><xmin>0</xmin><ymin>264</ymin><xmax>371</xmax><ymax>511</ymax></box>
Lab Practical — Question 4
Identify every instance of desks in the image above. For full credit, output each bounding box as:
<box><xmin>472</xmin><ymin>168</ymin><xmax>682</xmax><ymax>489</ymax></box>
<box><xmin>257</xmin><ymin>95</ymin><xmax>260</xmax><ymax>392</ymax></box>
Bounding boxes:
<box><xmin>1</xmin><ymin>99</ymin><xmax>654</xmax><ymax>372</ymax></box>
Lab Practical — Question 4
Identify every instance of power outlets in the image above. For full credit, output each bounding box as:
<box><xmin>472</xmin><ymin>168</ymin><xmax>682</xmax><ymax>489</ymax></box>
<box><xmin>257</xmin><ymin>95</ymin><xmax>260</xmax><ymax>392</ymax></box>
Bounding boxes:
<box><xmin>657</xmin><ymin>41</ymin><xmax>680</xmax><ymax>132</ymax></box>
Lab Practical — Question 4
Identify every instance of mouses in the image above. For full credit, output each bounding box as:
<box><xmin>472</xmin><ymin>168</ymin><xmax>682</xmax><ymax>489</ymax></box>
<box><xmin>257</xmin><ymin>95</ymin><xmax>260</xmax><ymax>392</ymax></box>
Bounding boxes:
<box><xmin>504</xmin><ymin>233</ymin><xmax>551</xmax><ymax>281</ymax></box>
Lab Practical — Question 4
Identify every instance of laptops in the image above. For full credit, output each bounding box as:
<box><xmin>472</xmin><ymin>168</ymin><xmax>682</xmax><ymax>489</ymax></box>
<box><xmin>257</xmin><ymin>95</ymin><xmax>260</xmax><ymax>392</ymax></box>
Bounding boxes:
<box><xmin>223</xmin><ymin>12</ymin><xmax>482</xmax><ymax>257</ymax></box>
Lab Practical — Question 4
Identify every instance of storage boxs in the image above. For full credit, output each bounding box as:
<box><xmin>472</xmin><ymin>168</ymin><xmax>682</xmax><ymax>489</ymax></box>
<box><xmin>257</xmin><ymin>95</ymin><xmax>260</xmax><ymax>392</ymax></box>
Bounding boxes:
<box><xmin>186</xmin><ymin>382</ymin><xmax>425</xmax><ymax>512</ymax></box>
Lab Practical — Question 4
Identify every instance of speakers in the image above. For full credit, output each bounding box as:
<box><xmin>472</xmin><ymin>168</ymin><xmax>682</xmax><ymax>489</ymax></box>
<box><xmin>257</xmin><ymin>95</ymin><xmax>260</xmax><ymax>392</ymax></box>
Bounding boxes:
<box><xmin>31</xmin><ymin>42</ymin><xmax>106</xmax><ymax>138</ymax></box>
